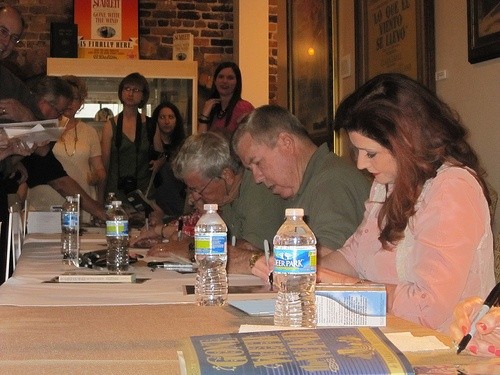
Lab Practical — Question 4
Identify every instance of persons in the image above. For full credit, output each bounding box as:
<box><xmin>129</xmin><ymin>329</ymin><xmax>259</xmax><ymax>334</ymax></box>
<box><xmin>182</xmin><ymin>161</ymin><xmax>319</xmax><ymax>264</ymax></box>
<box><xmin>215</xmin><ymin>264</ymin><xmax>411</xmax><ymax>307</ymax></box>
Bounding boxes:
<box><xmin>147</xmin><ymin>132</ymin><xmax>295</xmax><ymax>261</ymax></box>
<box><xmin>96</xmin><ymin>72</ymin><xmax>187</xmax><ymax>228</ymax></box>
<box><xmin>450</xmin><ymin>297</ymin><xmax>500</xmax><ymax>359</ymax></box>
<box><xmin>198</xmin><ymin>62</ymin><xmax>255</xmax><ymax>138</ymax></box>
<box><xmin>0</xmin><ymin>6</ymin><xmax>115</xmax><ymax>286</ymax></box>
<box><xmin>252</xmin><ymin>74</ymin><xmax>496</xmax><ymax>338</ymax></box>
<box><xmin>225</xmin><ymin>104</ymin><xmax>375</xmax><ymax>276</ymax></box>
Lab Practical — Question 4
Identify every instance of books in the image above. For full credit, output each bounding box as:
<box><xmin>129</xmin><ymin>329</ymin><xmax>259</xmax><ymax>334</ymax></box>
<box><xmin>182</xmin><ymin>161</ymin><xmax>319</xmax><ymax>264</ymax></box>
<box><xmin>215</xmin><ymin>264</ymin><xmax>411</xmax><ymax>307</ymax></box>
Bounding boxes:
<box><xmin>172</xmin><ymin>32</ymin><xmax>194</xmax><ymax>61</ymax></box>
<box><xmin>59</xmin><ymin>272</ymin><xmax>136</xmax><ymax>283</ymax></box>
<box><xmin>180</xmin><ymin>327</ymin><xmax>416</xmax><ymax>375</ymax></box>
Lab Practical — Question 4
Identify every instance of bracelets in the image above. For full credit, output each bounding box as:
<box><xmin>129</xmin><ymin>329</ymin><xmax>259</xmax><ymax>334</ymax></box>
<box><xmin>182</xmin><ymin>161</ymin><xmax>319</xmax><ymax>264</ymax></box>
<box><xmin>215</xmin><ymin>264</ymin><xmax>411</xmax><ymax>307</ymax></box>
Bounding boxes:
<box><xmin>200</xmin><ymin>114</ymin><xmax>210</xmax><ymax>119</ymax></box>
<box><xmin>199</xmin><ymin>118</ymin><xmax>209</xmax><ymax>124</ymax></box>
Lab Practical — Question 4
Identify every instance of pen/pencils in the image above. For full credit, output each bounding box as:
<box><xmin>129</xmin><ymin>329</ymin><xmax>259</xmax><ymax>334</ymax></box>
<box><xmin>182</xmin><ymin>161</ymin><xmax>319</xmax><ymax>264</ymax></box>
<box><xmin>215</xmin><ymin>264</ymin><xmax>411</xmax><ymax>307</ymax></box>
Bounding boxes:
<box><xmin>263</xmin><ymin>236</ymin><xmax>275</xmax><ymax>291</ymax></box>
<box><xmin>176</xmin><ymin>218</ymin><xmax>184</xmax><ymax>241</ymax></box>
<box><xmin>145</xmin><ymin>217</ymin><xmax>149</xmax><ymax>230</ymax></box>
<box><xmin>146</xmin><ymin>260</ymin><xmax>198</xmax><ymax>274</ymax></box>
<box><xmin>455</xmin><ymin>281</ymin><xmax>500</xmax><ymax>357</ymax></box>
<box><xmin>231</xmin><ymin>234</ymin><xmax>236</xmax><ymax>247</ymax></box>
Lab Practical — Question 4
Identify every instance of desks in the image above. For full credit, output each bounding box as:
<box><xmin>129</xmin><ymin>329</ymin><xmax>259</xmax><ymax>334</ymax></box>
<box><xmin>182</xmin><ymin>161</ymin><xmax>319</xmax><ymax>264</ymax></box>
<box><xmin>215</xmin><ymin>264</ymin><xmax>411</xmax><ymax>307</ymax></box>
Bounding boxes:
<box><xmin>0</xmin><ymin>227</ymin><xmax>499</xmax><ymax>375</ymax></box>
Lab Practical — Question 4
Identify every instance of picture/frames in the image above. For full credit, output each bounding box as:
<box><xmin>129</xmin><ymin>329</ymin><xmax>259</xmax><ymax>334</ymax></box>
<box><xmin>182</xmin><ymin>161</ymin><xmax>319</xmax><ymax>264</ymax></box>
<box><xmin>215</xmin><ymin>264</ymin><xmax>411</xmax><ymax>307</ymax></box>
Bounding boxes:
<box><xmin>467</xmin><ymin>0</ymin><xmax>500</xmax><ymax>65</ymax></box>
<box><xmin>286</xmin><ymin>0</ymin><xmax>333</xmax><ymax>145</ymax></box>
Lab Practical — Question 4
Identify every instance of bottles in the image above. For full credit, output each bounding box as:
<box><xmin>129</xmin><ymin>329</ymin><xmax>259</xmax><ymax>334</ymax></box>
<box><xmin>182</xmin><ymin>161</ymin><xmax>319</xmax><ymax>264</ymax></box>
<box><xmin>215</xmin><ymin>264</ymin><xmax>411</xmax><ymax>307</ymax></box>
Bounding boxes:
<box><xmin>106</xmin><ymin>193</ymin><xmax>118</xmax><ymax>211</ymax></box>
<box><xmin>272</xmin><ymin>208</ymin><xmax>318</xmax><ymax>330</ymax></box>
<box><xmin>193</xmin><ymin>204</ymin><xmax>228</xmax><ymax>308</ymax></box>
<box><xmin>106</xmin><ymin>201</ymin><xmax>130</xmax><ymax>275</ymax></box>
<box><xmin>60</xmin><ymin>195</ymin><xmax>78</xmax><ymax>258</ymax></box>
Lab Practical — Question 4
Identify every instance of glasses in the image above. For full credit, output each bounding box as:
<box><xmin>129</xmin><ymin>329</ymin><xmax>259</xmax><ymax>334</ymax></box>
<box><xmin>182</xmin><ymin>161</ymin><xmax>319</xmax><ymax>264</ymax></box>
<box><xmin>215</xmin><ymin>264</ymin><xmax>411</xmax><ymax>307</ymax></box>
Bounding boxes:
<box><xmin>122</xmin><ymin>86</ymin><xmax>143</xmax><ymax>94</ymax></box>
<box><xmin>43</xmin><ymin>98</ymin><xmax>65</xmax><ymax>118</ymax></box>
<box><xmin>0</xmin><ymin>27</ymin><xmax>23</xmax><ymax>44</ymax></box>
<box><xmin>71</xmin><ymin>95</ymin><xmax>84</xmax><ymax>105</ymax></box>
<box><xmin>100</xmin><ymin>108</ymin><xmax>110</xmax><ymax>115</ymax></box>
<box><xmin>159</xmin><ymin>114</ymin><xmax>175</xmax><ymax>120</ymax></box>
<box><xmin>180</xmin><ymin>176</ymin><xmax>216</xmax><ymax>200</ymax></box>
